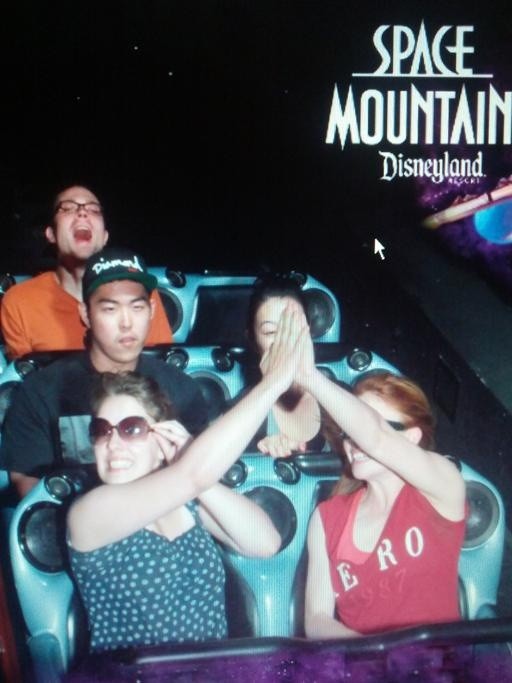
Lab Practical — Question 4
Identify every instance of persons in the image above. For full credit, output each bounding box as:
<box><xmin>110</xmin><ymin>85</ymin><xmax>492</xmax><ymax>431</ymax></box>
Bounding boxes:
<box><xmin>0</xmin><ymin>246</ymin><xmax>210</xmax><ymax>508</ymax></box>
<box><xmin>0</xmin><ymin>183</ymin><xmax>174</xmax><ymax>365</ymax></box>
<box><xmin>296</xmin><ymin>302</ymin><xmax>473</xmax><ymax>643</ymax></box>
<box><xmin>219</xmin><ymin>265</ymin><xmax>355</xmax><ymax>460</ymax></box>
<box><xmin>66</xmin><ymin>299</ymin><xmax>311</xmax><ymax>658</ymax></box>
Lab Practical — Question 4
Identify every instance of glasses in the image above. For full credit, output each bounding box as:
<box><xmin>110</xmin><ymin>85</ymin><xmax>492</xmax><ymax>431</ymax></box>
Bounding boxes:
<box><xmin>53</xmin><ymin>200</ymin><xmax>103</xmax><ymax>217</ymax></box>
<box><xmin>340</xmin><ymin>421</ymin><xmax>406</xmax><ymax>438</ymax></box>
<box><xmin>87</xmin><ymin>416</ymin><xmax>154</xmax><ymax>444</ymax></box>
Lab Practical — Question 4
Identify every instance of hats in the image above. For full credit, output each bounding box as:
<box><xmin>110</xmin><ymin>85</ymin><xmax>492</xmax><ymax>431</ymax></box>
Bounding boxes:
<box><xmin>82</xmin><ymin>248</ymin><xmax>156</xmax><ymax>301</ymax></box>
